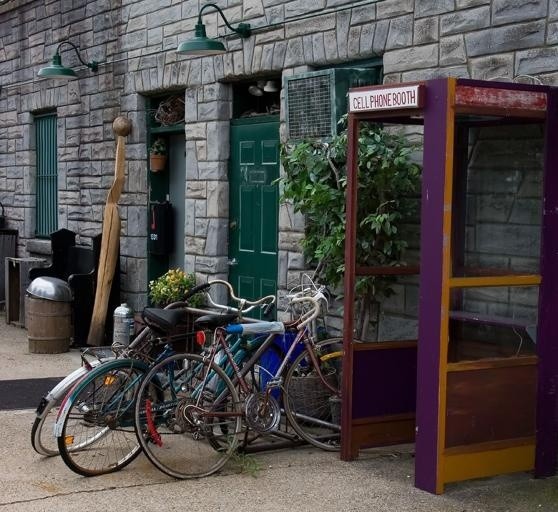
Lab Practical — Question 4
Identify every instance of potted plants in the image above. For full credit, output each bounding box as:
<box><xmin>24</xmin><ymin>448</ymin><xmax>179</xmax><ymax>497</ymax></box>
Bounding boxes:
<box><xmin>148</xmin><ymin>139</ymin><xmax>168</xmax><ymax>173</ymax></box>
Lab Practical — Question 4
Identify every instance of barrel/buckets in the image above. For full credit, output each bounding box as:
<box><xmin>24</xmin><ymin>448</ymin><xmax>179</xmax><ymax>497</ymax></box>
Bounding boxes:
<box><xmin>26</xmin><ymin>295</ymin><xmax>72</xmax><ymax>355</ymax></box>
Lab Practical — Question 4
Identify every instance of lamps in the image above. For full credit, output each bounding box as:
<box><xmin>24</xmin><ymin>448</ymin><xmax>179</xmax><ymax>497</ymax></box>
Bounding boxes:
<box><xmin>264</xmin><ymin>81</ymin><xmax>278</xmax><ymax>92</ymax></box>
<box><xmin>175</xmin><ymin>4</ymin><xmax>251</xmax><ymax>55</ymax></box>
<box><xmin>248</xmin><ymin>80</ymin><xmax>265</xmax><ymax>97</ymax></box>
<box><xmin>37</xmin><ymin>42</ymin><xmax>98</xmax><ymax>79</ymax></box>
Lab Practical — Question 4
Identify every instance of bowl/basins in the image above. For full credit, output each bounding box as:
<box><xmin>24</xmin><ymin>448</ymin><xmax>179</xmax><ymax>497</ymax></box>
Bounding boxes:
<box><xmin>26</xmin><ymin>276</ymin><xmax>74</xmax><ymax>304</ymax></box>
